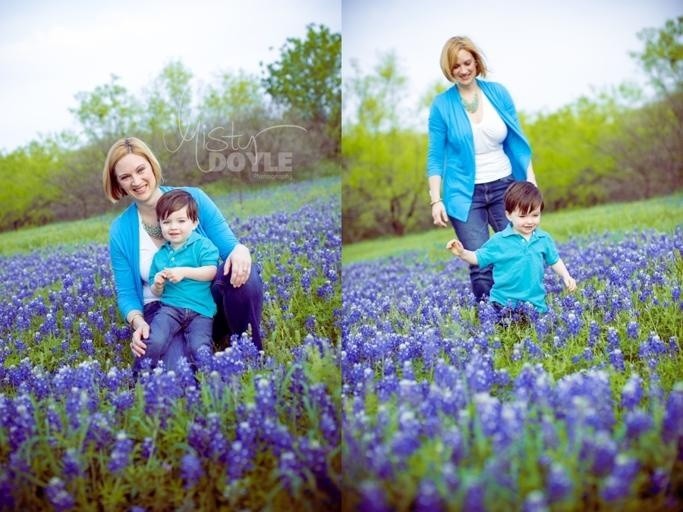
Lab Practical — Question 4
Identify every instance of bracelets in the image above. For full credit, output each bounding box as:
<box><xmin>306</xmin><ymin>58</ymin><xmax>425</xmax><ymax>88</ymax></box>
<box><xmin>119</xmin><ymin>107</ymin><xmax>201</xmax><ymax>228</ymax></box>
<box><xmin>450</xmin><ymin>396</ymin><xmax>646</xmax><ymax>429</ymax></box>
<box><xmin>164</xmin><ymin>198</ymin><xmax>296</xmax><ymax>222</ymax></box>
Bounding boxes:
<box><xmin>130</xmin><ymin>314</ymin><xmax>144</xmax><ymax>333</ymax></box>
<box><xmin>430</xmin><ymin>199</ymin><xmax>443</xmax><ymax>207</ymax></box>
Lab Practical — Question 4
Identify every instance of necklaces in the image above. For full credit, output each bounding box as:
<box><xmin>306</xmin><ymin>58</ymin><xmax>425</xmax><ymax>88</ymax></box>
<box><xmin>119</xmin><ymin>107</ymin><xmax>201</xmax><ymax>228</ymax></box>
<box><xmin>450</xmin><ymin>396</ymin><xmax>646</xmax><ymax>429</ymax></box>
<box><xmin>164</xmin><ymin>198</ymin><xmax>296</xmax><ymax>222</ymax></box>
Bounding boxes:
<box><xmin>139</xmin><ymin>214</ymin><xmax>163</xmax><ymax>240</ymax></box>
<box><xmin>458</xmin><ymin>81</ymin><xmax>479</xmax><ymax>113</ymax></box>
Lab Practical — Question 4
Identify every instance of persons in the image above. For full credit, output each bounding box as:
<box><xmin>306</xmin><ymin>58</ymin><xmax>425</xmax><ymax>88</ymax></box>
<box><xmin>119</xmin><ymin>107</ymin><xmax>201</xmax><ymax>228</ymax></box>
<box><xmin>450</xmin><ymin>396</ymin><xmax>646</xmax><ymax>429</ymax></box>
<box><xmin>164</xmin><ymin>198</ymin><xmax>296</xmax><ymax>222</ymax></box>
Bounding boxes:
<box><xmin>425</xmin><ymin>36</ymin><xmax>537</xmax><ymax>332</ymax></box>
<box><xmin>102</xmin><ymin>136</ymin><xmax>263</xmax><ymax>398</ymax></box>
<box><xmin>126</xmin><ymin>189</ymin><xmax>220</xmax><ymax>389</ymax></box>
<box><xmin>445</xmin><ymin>181</ymin><xmax>578</xmax><ymax>333</ymax></box>
